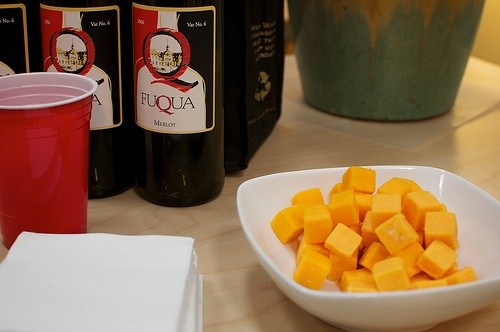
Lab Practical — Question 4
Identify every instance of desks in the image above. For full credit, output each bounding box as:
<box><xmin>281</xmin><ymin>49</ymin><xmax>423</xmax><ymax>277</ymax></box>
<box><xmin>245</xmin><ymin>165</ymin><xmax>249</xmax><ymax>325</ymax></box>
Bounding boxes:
<box><xmin>0</xmin><ymin>56</ymin><xmax>500</xmax><ymax>332</ymax></box>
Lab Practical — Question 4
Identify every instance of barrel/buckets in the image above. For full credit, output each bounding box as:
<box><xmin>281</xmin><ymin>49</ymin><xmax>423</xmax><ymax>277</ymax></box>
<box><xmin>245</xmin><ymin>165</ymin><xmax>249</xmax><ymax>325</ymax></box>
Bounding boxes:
<box><xmin>287</xmin><ymin>0</ymin><xmax>485</xmax><ymax>121</ymax></box>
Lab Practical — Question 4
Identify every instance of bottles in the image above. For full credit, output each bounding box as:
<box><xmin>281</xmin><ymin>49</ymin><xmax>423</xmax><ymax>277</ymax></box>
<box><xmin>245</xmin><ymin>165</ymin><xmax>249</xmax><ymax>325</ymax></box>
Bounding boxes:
<box><xmin>40</xmin><ymin>0</ymin><xmax>133</xmax><ymax>198</ymax></box>
<box><xmin>130</xmin><ymin>0</ymin><xmax>225</xmax><ymax>206</ymax></box>
<box><xmin>0</xmin><ymin>0</ymin><xmax>40</xmax><ymax>78</ymax></box>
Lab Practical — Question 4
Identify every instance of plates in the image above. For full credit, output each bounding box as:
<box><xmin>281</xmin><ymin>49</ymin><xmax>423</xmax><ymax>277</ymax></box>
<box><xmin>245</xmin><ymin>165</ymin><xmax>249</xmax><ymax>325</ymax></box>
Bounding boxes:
<box><xmin>236</xmin><ymin>165</ymin><xmax>500</xmax><ymax>332</ymax></box>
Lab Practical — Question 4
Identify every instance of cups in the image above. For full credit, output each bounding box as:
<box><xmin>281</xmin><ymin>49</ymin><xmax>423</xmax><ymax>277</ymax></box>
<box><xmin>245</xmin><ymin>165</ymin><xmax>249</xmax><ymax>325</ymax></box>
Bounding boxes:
<box><xmin>0</xmin><ymin>72</ymin><xmax>98</xmax><ymax>250</ymax></box>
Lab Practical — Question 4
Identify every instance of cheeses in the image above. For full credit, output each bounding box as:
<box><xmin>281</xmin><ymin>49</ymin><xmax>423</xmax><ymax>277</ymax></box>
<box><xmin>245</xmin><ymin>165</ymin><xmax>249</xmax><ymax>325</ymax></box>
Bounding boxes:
<box><xmin>271</xmin><ymin>165</ymin><xmax>476</xmax><ymax>292</ymax></box>
